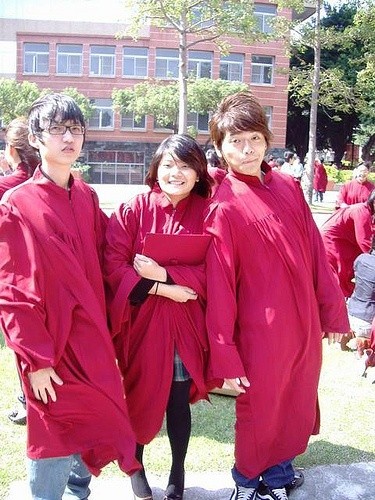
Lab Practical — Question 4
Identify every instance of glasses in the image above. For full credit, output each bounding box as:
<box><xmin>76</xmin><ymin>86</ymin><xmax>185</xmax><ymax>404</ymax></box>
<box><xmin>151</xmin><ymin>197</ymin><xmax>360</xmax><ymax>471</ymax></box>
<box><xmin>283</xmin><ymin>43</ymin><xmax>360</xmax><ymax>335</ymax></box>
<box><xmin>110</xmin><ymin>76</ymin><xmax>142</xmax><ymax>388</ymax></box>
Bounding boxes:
<box><xmin>41</xmin><ymin>123</ymin><xmax>85</xmax><ymax>135</ymax></box>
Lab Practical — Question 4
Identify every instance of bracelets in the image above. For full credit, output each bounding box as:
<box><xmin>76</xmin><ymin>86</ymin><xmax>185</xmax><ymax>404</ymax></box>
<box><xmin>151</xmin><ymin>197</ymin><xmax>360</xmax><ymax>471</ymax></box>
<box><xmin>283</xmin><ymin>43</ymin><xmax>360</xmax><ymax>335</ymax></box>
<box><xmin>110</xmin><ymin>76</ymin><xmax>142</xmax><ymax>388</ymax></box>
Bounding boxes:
<box><xmin>155</xmin><ymin>282</ymin><xmax>160</xmax><ymax>295</ymax></box>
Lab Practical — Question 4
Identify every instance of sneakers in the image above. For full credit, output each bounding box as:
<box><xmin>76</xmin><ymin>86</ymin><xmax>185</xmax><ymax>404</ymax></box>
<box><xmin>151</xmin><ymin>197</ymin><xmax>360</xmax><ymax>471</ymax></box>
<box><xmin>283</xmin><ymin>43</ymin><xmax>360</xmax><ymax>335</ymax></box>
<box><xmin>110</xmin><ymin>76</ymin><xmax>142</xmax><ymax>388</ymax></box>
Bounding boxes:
<box><xmin>256</xmin><ymin>480</ymin><xmax>288</xmax><ymax>500</ymax></box>
<box><xmin>228</xmin><ymin>483</ymin><xmax>256</xmax><ymax>500</ymax></box>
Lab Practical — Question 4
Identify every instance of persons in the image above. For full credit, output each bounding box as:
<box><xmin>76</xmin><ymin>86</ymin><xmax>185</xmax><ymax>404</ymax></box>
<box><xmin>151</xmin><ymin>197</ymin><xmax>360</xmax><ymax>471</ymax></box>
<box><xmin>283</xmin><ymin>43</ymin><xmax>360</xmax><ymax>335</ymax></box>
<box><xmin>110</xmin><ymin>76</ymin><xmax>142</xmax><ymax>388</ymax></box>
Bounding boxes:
<box><xmin>267</xmin><ymin>151</ymin><xmax>328</xmax><ymax>202</ymax></box>
<box><xmin>335</xmin><ymin>165</ymin><xmax>375</xmax><ymax>209</ymax></box>
<box><xmin>0</xmin><ymin>93</ymin><xmax>143</xmax><ymax>500</ymax></box>
<box><xmin>104</xmin><ymin>135</ymin><xmax>212</xmax><ymax>500</ymax></box>
<box><xmin>0</xmin><ymin>115</ymin><xmax>40</xmax><ymax>426</ymax></box>
<box><xmin>318</xmin><ymin>191</ymin><xmax>375</xmax><ymax>299</ymax></box>
<box><xmin>205</xmin><ymin>92</ymin><xmax>350</xmax><ymax>500</ymax></box>
<box><xmin>340</xmin><ymin>236</ymin><xmax>375</xmax><ymax>351</ymax></box>
<box><xmin>205</xmin><ymin>148</ymin><xmax>229</xmax><ymax>198</ymax></box>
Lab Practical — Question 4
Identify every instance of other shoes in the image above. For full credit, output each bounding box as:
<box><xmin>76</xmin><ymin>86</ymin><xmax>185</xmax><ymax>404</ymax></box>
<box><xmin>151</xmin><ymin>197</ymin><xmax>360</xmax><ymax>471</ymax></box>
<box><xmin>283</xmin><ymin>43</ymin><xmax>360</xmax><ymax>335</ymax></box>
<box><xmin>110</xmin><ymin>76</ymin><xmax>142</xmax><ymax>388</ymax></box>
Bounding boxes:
<box><xmin>163</xmin><ymin>467</ymin><xmax>184</xmax><ymax>499</ymax></box>
<box><xmin>131</xmin><ymin>469</ymin><xmax>152</xmax><ymax>500</ymax></box>
<box><xmin>19</xmin><ymin>396</ymin><xmax>25</xmax><ymax>404</ymax></box>
<box><xmin>288</xmin><ymin>470</ymin><xmax>304</xmax><ymax>489</ymax></box>
<box><xmin>8</xmin><ymin>411</ymin><xmax>28</xmax><ymax>424</ymax></box>
